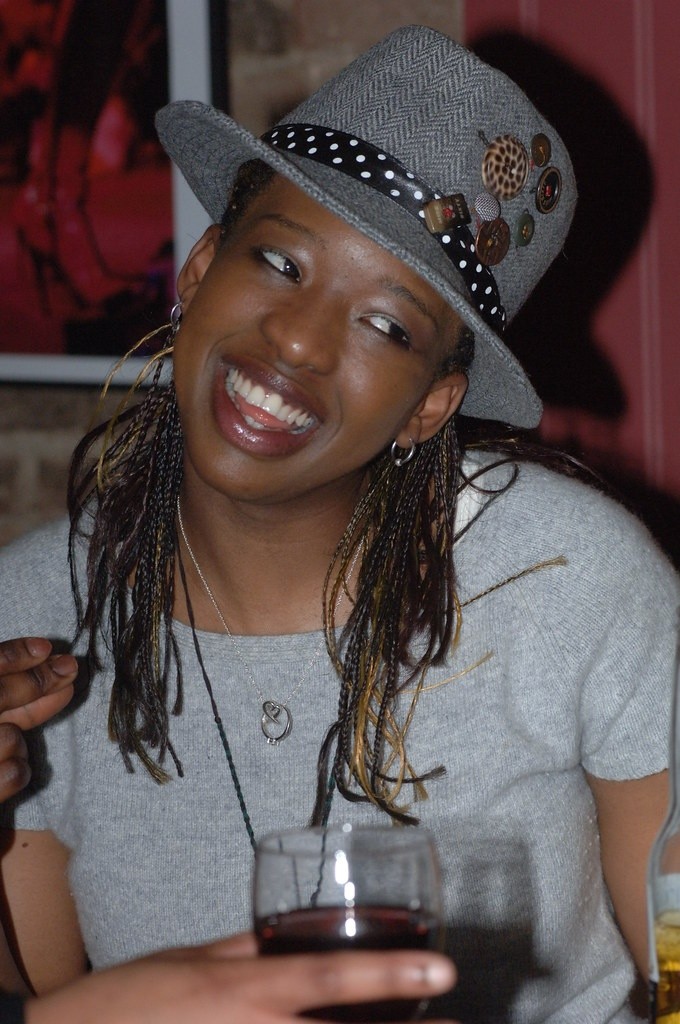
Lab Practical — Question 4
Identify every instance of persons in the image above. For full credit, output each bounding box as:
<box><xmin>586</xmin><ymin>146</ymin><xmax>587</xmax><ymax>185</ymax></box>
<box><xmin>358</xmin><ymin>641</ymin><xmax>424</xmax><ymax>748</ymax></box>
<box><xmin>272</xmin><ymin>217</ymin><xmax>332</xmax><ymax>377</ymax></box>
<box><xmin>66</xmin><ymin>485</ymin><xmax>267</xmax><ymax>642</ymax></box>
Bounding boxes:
<box><xmin>0</xmin><ymin>22</ymin><xmax>680</xmax><ymax>1024</ymax></box>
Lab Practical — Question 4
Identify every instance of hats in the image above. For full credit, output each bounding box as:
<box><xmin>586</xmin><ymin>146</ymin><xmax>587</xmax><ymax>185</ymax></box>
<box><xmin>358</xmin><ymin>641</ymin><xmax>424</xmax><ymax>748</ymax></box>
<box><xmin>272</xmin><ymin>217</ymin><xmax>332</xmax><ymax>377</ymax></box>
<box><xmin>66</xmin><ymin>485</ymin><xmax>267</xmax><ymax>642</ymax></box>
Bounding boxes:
<box><xmin>155</xmin><ymin>23</ymin><xmax>577</xmax><ymax>427</ymax></box>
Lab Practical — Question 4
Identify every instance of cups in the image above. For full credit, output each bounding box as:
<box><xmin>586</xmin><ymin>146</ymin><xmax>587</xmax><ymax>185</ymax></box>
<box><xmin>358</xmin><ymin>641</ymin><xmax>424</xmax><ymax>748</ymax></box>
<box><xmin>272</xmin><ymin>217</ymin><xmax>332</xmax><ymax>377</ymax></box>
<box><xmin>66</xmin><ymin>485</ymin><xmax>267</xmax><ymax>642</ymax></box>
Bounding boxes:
<box><xmin>252</xmin><ymin>823</ymin><xmax>446</xmax><ymax>1024</ymax></box>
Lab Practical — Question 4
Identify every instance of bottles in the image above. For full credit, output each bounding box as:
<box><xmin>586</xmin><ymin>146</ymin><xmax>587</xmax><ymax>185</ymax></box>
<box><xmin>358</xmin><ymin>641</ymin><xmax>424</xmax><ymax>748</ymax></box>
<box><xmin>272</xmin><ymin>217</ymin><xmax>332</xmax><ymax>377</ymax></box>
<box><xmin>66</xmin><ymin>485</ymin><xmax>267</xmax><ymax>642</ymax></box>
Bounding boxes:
<box><xmin>645</xmin><ymin>605</ymin><xmax>680</xmax><ymax>1024</ymax></box>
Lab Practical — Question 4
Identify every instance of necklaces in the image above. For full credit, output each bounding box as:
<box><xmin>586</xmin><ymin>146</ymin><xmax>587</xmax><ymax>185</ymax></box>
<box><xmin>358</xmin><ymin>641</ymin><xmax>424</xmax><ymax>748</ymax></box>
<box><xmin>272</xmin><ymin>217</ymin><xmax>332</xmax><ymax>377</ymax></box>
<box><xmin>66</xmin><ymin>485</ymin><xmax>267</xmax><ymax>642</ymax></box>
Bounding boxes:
<box><xmin>175</xmin><ymin>496</ymin><xmax>364</xmax><ymax>744</ymax></box>
<box><xmin>172</xmin><ymin>531</ymin><xmax>331</xmax><ymax>911</ymax></box>
